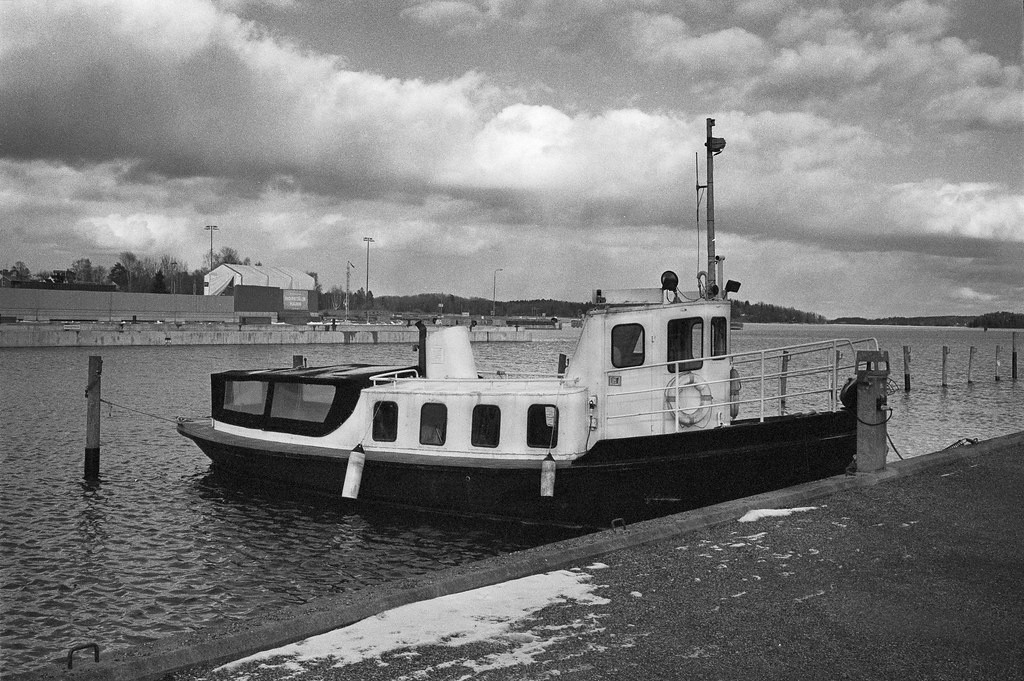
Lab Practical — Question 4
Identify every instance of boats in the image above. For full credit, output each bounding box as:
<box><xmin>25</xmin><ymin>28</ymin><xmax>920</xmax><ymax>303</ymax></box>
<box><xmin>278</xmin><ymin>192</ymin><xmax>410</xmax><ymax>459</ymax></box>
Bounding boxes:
<box><xmin>175</xmin><ymin>116</ymin><xmax>883</xmax><ymax>520</ymax></box>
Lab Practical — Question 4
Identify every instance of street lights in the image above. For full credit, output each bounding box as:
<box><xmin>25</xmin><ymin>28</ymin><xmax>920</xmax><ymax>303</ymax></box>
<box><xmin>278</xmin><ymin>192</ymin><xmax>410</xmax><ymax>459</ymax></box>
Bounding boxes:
<box><xmin>204</xmin><ymin>224</ymin><xmax>219</xmax><ymax>271</ymax></box>
<box><xmin>493</xmin><ymin>269</ymin><xmax>504</xmax><ymax>317</ymax></box>
<box><xmin>363</xmin><ymin>236</ymin><xmax>376</xmax><ymax>318</ymax></box>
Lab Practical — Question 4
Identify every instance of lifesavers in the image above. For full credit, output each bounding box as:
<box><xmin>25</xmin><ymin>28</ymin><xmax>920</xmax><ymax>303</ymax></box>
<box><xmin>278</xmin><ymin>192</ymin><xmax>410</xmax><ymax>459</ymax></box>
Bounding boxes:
<box><xmin>667</xmin><ymin>374</ymin><xmax>712</xmax><ymax>425</ymax></box>
<box><xmin>730</xmin><ymin>368</ymin><xmax>741</xmax><ymax>418</ymax></box>
